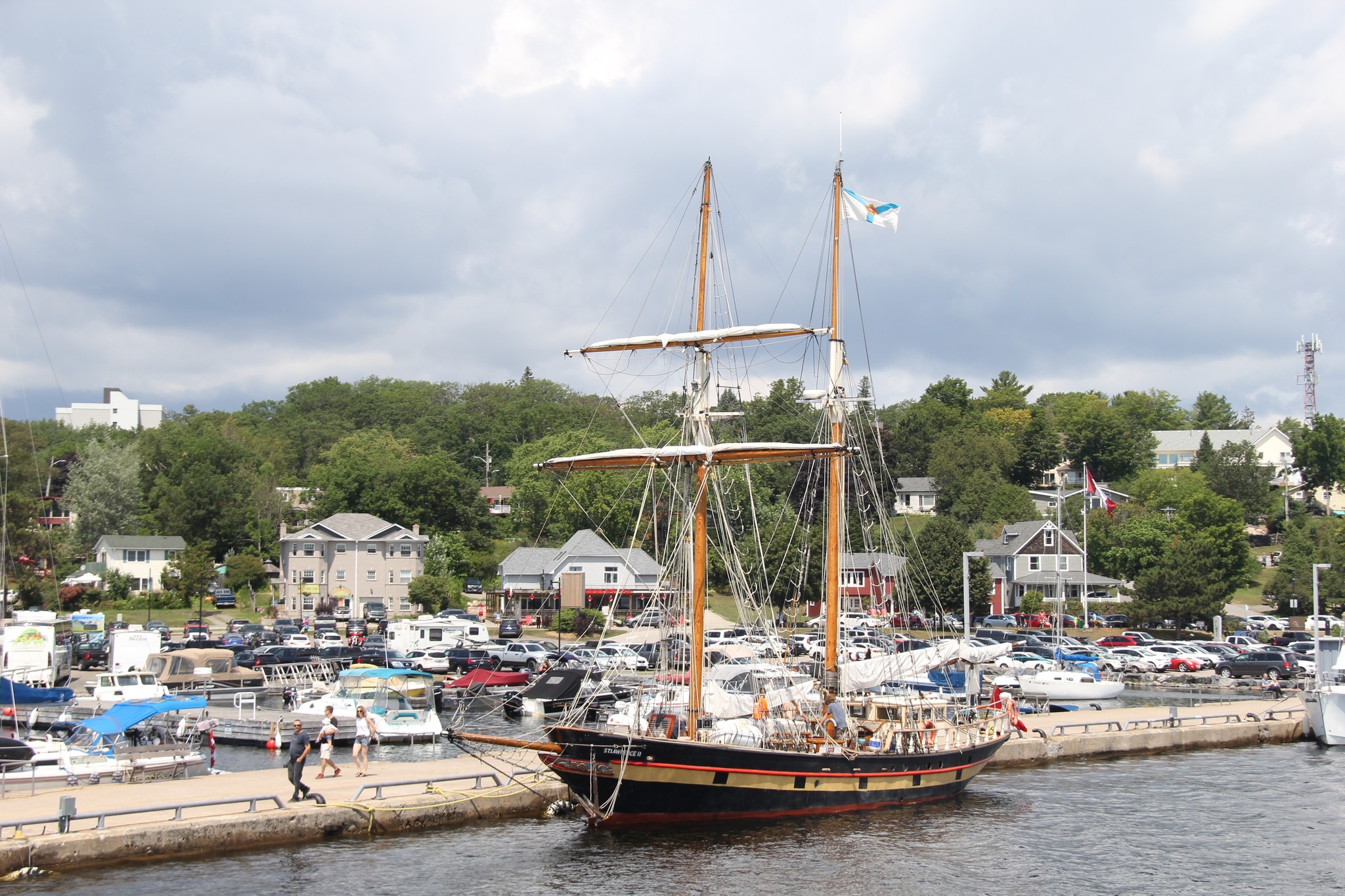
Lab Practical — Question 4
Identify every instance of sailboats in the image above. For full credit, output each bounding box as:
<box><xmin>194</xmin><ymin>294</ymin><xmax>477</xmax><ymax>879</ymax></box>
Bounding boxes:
<box><xmin>435</xmin><ymin>162</ymin><xmax>1033</xmax><ymax>846</ymax></box>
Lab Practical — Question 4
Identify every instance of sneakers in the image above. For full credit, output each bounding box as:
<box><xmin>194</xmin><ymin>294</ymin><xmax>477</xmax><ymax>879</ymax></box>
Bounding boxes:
<box><xmin>315</xmin><ymin>773</ymin><xmax>324</xmax><ymax>779</ymax></box>
<box><xmin>334</xmin><ymin>768</ymin><xmax>341</xmax><ymax>777</ymax></box>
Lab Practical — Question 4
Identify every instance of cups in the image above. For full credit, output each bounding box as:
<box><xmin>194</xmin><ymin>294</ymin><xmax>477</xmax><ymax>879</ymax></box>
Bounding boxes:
<box><xmin>1271</xmin><ymin>682</ymin><xmax>1273</xmax><ymax>684</ymax></box>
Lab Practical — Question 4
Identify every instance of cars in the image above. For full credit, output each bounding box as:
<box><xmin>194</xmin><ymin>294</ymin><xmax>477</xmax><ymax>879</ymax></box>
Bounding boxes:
<box><xmin>0</xmin><ymin>588</ymin><xmax>21</xmax><ymax>606</ymax></box>
<box><xmin>332</xmin><ymin>605</ymin><xmax>352</xmax><ymax>622</ymax></box>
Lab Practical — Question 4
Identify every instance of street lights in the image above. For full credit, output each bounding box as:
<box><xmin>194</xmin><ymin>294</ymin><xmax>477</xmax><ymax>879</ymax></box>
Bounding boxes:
<box><xmin>547</xmin><ymin>577</ymin><xmax>561</xmax><ymax>654</ymax></box>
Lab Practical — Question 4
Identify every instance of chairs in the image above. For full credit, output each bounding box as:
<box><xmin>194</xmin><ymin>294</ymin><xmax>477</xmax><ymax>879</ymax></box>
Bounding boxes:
<box><xmin>1257</xmin><ymin>682</ymin><xmax>1274</xmax><ymax>700</ymax></box>
<box><xmin>851</xmin><ymin>719</ymin><xmax>887</xmax><ymax>751</ymax></box>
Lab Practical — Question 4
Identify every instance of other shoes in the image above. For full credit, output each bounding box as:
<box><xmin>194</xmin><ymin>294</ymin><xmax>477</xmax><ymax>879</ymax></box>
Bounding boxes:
<box><xmin>1039</xmin><ymin>626</ymin><xmax>1043</xmax><ymax>629</ymax></box>
<box><xmin>1050</xmin><ymin>629</ymin><xmax>1052</xmax><ymax>629</ymax></box>
<box><xmin>1020</xmin><ymin>627</ymin><xmax>1022</xmax><ymax>630</ymax></box>
<box><xmin>303</xmin><ymin>787</ymin><xmax>310</xmax><ymax>799</ymax></box>
<box><xmin>1275</xmin><ymin>698</ymin><xmax>1279</xmax><ymax>701</ymax></box>
<box><xmin>315</xmin><ymin>739</ymin><xmax>320</xmax><ymax>744</ymax></box>
<box><xmin>1054</xmin><ymin>628</ymin><xmax>1056</xmax><ymax>629</ymax></box>
<box><xmin>329</xmin><ymin>745</ymin><xmax>334</xmax><ymax>751</ymax></box>
<box><xmin>288</xmin><ymin>795</ymin><xmax>299</xmax><ymax>802</ymax></box>
<box><xmin>1281</xmin><ymin>694</ymin><xmax>1287</xmax><ymax>698</ymax></box>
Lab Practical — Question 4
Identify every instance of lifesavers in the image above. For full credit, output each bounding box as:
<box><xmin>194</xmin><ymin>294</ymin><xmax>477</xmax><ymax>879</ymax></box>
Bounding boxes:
<box><xmin>1007</xmin><ymin>699</ymin><xmax>1018</xmax><ymax>725</ymax></box>
<box><xmin>916</xmin><ymin>719</ymin><xmax>937</xmax><ymax>748</ymax></box>
<box><xmin>1099</xmin><ymin>670</ymin><xmax>1104</xmax><ymax>681</ymax></box>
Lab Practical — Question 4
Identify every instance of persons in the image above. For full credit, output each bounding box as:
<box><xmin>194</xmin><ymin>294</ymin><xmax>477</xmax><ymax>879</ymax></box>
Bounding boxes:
<box><xmin>670</xmin><ymin>715</ymin><xmax>688</xmax><ymax>739</ymax></box>
<box><xmin>1054</xmin><ymin>613</ymin><xmax>1058</xmax><ymax>628</ymax></box>
<box><xmin>1014</xmin><ymin>611</ymin><xmax>1022</xmax><ymax>630</ymax></box>
<box><xmin>303</xmin><ymin>618</ymin><xmax>309</xmax><ymax>634</ymax></box>
<box><xmin>1262</xmin><ymin>673</ymin><xmax>1287</xmax><ymax>701</ymax></box>
<box><xmin>1264</xmin><ymin>626</ymin><xmax>1270</xmax><ymax>634</ymax></box>
<box><xmin>1048</xmin><ymin>611</ymin><xmax>1054</xmax><ymax>629</ymax></box>
<box><xmin>1073</xmin><ymin>615</ymin><xmax>1078</xmax><ymax>629</ymax></box>
<box><xmin>1271</xmin><ymin>678</ymin><xmax>1279</xmax><ymax>687</ymax></box>
<box><xmin>1038</xmin><ymin>610</ymin><xmax>1046</xmax><ymax>629</ymax></box>
<box><xmin>285</xmin><ymin>719</ymin><xmax>311</xmax><ymax>802</ymax></box>
<box><xmin>315</xmin><ymin>705</ymin><xmax>342</xmax><ymax>779</ymax></box>
<box><xmin>540</xmin><ymin>662</ymin><xmax>550</xmax><ymax>674</ymax></box>
<box><xmin>1093</xmin><ymin>611</ymin><xmax>1096</xmax><ymax>627</ymax></box>
<box><xmin>972</xmin><ymin>614</ymin><xmax>976</xmax><ymax>630</ymax></box>
<box><xmin>1020</xmin><ymin>612</ymin><xmax>1027</xmax><ymax>627</ymax></box>
<box><xmin>775</xmin><ymin>611</ymin><xmax>787</xmax><ymax>628</ymax></box>
<box><xmin>315</xmin><ymin>718</ymin><xmax>340</xmax><ymax>752</ymax></box>
<box><xmin>782</xmin><ymin>699</ymin><xmax>809</xmax><ymax>722</ymax></box>
<box><xmin>816</xmin><ymin>693</ymin><xmax>847</xmax><ymax>740</ymax></box>
<box><xmin>752</xmin><ymin>688</ymin><xmax>772</xmax><ymax>738</ymax></box>
<box><xmin>916</xmin><ymin>616</ymin><xmax>921</xmax><ymax>628</ymax></box>
<box><xmin>1246</xmin><ymin>623</ymin><xmax>1252</xmax><ymax>630</ymax></box>
<box><xmin>352</xmin><ymin>706</ymin><xmax>376</xmax><ymax>777</ymax></box>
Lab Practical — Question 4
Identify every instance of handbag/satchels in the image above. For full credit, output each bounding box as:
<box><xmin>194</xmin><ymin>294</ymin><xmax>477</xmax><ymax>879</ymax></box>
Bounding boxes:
<box><xmin>305</xmin><ymin>625</ymin><xmax>309</xmax><ymax>629</ymax></box>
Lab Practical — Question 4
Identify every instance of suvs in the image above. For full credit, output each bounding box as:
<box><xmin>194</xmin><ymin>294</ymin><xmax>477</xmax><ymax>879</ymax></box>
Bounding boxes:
<box><xmin>226</xmin><ymin>618</ymin><xmax>250</xmax><ymax>632</ymax></box>
<box><xmin>377</xmin><ymin>619</ymin><xmax>397</xmax><ymax>632</ymax></box>
<box><xmin>433</xmin><ymin>607</ymin><xmax>468</xmax><ymax>618</ymax></box>
<box><xmin>498</xmin><ymin>618</ymin><xmax>522</xmax><ymax>638</ymax></box>
<box><xmin>623</xmin><ymin>611</ymin><xmax>668</xmax><ymax>629</ymax></box>
<box><xmin>363</xmin><ymin>601</ymin><xmax>388</xmax><ymax>623</ymax></box>
<box><xmin>463</xmin><ymin>578</ymin><xmax>483</xmax><ymax>594</ymax></box>
<box><xmin>344</xmin><ymin>617</ymin><xmax>369</xmax><ymax>638</ymax></box>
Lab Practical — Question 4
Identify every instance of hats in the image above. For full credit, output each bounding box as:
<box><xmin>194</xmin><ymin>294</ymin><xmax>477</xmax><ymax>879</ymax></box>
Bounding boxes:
<box><xmin>322</xmin><ymin>718</ymin><xmax>331</xmax><ymax>725</ymax></box>
<box><xmin>1022</xmin><ymin>612</ymin><xmax>1025</xmax><ymax>614</ymax></box>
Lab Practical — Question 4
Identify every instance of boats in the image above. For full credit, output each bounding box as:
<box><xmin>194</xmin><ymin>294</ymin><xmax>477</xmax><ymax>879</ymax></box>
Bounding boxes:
<box><xmin>0</xmin><ymin>613</ymin><xmax>1345</xmax><ymax>781</ymax></box>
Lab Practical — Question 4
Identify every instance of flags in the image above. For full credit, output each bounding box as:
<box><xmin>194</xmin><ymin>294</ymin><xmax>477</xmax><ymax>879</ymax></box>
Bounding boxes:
<box><xmin>1085</xmin><ymin>466</ymin><xmax>1117</xmax><ymax>519</ymax></box>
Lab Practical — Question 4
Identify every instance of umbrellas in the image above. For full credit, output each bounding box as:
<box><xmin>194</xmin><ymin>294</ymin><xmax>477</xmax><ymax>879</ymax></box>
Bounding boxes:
<box><xmin>478</xmin><ymin>603</ymin><xmax>483</xmax><ymax>621</ymax></box>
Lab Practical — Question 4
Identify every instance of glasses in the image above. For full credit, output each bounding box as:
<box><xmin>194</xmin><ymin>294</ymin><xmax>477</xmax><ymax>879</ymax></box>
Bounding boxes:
<box><xmin>294</xmin><ymin>725</ymin><xmax>300</xmax><ymax>727</ymax></box>
<box><xmin>357</xmin><ymin>710</ymin><xmax>362</xmax><ymax>713</ymax></box>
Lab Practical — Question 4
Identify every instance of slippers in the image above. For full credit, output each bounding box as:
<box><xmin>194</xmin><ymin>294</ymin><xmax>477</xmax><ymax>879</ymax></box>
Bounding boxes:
<box><xmin>355</xmin><ymin>772</ymin><xmax>367</xmax><ymax>777</ymax></box>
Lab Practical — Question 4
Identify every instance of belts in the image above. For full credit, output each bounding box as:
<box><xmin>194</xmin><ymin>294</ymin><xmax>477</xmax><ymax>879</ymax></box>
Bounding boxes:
<box><xmin>358</xmin><ymin>735</ymin><xmax>367</xmax><ymax>738</ymax></box>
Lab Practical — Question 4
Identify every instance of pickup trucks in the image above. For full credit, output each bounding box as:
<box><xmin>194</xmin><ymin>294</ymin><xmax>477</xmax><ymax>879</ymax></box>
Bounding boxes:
<box><xmin>313</xmin><ymin>615</ymin><xmax>339</xmax><ymax>630</ymax></box>
<box><xmin>212</xmin><ymin>588</ymin><xmax>237</xmax><ymax>609</ymax></box>
